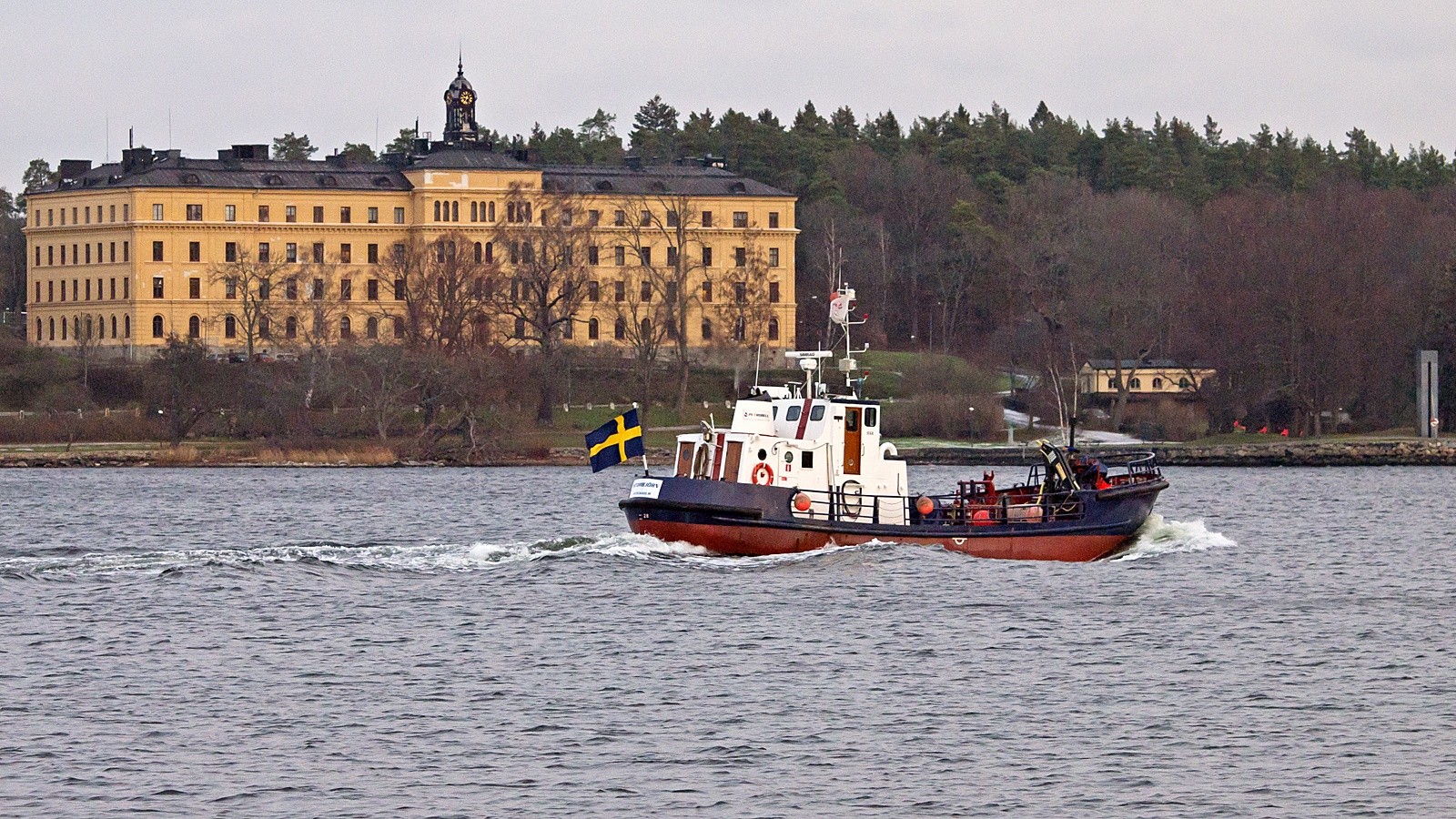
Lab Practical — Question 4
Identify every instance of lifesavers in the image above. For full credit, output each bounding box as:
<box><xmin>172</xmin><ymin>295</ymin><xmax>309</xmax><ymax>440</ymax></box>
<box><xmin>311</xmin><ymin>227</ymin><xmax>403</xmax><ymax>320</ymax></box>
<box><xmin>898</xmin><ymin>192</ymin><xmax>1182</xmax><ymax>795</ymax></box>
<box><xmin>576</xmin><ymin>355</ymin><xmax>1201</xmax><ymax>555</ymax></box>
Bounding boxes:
<box><xmin>752</xmin><ymin>462</ymin><xmax>774</xmax><ymax>486</ymax></box>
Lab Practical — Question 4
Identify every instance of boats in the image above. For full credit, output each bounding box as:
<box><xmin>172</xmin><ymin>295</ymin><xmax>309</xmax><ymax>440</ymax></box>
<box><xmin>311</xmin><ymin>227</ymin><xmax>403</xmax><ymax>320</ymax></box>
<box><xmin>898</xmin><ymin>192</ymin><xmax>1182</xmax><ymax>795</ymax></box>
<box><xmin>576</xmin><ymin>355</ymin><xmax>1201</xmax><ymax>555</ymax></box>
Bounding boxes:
<box><xmin>618</xmin><ymin>246</ymin><xmax>1168</xmax><ymax>563</ymax></box>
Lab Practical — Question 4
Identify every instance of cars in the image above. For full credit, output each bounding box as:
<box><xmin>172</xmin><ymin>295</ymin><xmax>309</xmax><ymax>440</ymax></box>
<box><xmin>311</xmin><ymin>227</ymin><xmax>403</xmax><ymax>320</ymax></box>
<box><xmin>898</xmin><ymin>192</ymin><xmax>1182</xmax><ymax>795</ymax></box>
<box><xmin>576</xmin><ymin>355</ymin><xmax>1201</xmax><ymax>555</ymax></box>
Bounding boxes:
<box><xmin>207</xmin><ymin>352</ymin><xmax>297</xmax><ymax>363</ymax></box>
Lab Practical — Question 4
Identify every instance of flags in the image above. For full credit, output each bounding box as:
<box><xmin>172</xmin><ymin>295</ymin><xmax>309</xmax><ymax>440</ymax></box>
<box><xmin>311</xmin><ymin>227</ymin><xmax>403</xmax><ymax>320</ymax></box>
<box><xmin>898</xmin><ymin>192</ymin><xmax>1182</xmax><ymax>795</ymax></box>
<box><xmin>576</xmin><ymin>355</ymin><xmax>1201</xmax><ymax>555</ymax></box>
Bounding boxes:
<box><xmin>585</xmin><ymin>408</ymin><xmax>645</xmax><ymax>473</ymax></box>
